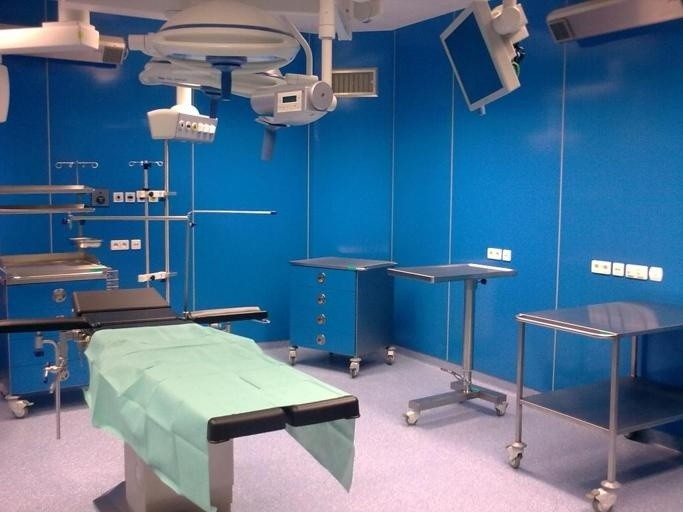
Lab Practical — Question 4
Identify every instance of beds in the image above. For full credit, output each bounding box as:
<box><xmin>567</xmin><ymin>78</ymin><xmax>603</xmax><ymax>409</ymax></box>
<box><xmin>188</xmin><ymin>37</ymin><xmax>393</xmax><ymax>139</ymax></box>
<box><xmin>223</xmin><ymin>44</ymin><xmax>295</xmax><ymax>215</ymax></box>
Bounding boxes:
<box><xmin>71</xmin><ymin>287</ymin><xmax>360</xmax><ymax>512</ymax></box>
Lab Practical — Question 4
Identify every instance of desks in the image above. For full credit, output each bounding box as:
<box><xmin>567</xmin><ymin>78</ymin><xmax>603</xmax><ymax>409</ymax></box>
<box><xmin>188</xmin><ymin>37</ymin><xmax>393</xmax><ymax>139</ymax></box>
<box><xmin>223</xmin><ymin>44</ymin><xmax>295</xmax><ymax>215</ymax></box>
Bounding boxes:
<box><xmin>505</xmin><ymin>301</ymin><xmax>683</xmax><ymax>512</ymax></box>
<box><xmin>387</xmin><ymin>263</ymin><xmax>517</xmax><ymax>426</ymax></box>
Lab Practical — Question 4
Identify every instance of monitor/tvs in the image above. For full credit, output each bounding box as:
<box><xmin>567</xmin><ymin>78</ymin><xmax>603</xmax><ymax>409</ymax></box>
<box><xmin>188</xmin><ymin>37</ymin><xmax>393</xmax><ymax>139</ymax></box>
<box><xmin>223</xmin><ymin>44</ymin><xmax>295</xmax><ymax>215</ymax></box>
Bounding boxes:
<box><xmin>439</xmin><ymin>0</ymin><xmax>521</xmax><ymax>112</ymax></box>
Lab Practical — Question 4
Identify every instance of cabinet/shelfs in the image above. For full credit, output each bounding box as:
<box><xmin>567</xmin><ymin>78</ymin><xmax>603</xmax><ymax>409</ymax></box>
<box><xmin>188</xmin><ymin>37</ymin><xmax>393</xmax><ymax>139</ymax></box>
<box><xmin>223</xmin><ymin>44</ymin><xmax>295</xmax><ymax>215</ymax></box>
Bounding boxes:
<box><xmin>0</xmin><ymin>251</ymin><xmax>108</xmax><ymax>419</ymax></box>
<box><xmin>288</xmin><ymin>256</ymin><xmax>397</xmax><ymax>378</ymax></box>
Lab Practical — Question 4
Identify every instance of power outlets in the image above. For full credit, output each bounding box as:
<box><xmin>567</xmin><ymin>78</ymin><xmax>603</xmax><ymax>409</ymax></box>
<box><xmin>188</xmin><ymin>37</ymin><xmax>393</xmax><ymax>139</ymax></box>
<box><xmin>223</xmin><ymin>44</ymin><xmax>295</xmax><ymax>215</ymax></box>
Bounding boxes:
<box><xmin>111</xmin><ymin>239</ymin><xmax>141</xmax><ymax>250</ymax></box>
<box><xmin>487</xmin><ymin>248</ymin><xmax>512</xmax><ymax>262</ymax></box>
<box><xmin>113</xmin><ymin>191</ymin><xmax>158</xmax><ymax>203</ymax></box>
